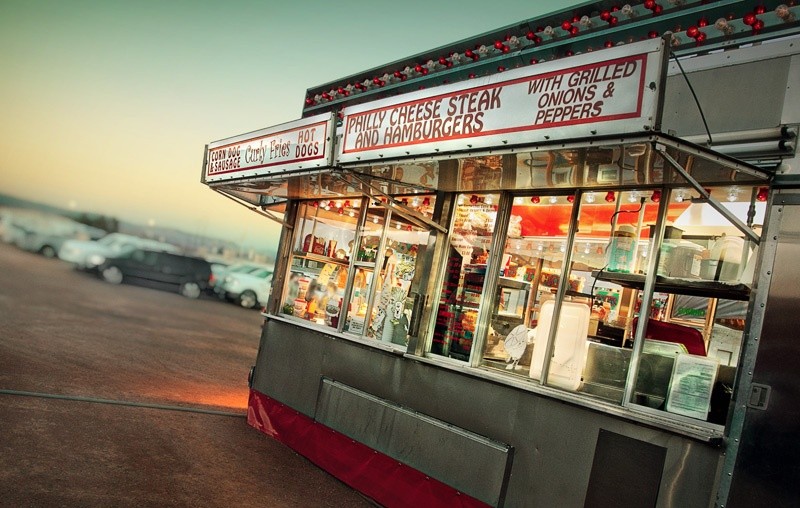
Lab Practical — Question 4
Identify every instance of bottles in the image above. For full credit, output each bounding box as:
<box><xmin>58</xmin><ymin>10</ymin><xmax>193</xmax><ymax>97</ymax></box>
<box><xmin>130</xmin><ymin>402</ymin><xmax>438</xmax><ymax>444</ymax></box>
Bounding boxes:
<box><xmin>606</xmin><ymin>226</ymin><xmax>636</xmax><ymax>272</ymax></box>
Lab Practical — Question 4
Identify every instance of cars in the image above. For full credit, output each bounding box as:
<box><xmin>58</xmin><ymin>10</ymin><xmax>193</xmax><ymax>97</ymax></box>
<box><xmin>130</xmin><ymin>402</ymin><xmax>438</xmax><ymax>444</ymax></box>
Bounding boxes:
<box><xmin>201</xmin><ymin>255</ymin><xmax>305</xmax><ymax>310</ymax></box>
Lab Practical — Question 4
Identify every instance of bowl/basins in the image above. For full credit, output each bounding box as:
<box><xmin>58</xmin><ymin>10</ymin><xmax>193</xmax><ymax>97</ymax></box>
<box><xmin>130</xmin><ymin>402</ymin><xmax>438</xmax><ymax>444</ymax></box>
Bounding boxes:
<box><xmin>700</xmin><ymin>259</ymin><xmax>739</xmax><ymax>281</ymax></box>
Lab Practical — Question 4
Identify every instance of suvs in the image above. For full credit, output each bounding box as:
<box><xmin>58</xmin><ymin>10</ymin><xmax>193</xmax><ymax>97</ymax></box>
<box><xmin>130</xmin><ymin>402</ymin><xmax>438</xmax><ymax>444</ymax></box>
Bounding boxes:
<box><xmin>88</xmin><ymin>249</ymin><xmax>217</xmax><ymax>299</ymax></box>
<box><xmin>1</xmin><ymin>209</ymin><xmax>182</xmax><ymax>273</ymax></box>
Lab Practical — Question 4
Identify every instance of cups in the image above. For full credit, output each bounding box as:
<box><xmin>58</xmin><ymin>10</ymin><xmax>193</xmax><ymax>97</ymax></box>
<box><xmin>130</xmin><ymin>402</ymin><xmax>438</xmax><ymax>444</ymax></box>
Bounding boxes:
<box><xmin>297</xmin><ymin>279</ymin><xmax>309</xmax><ymax>298</ymax></box>
<box><xmin>295</xmin><ymin>299</ymin><xmax>307</xmax><ymax>318</ymax></box>
